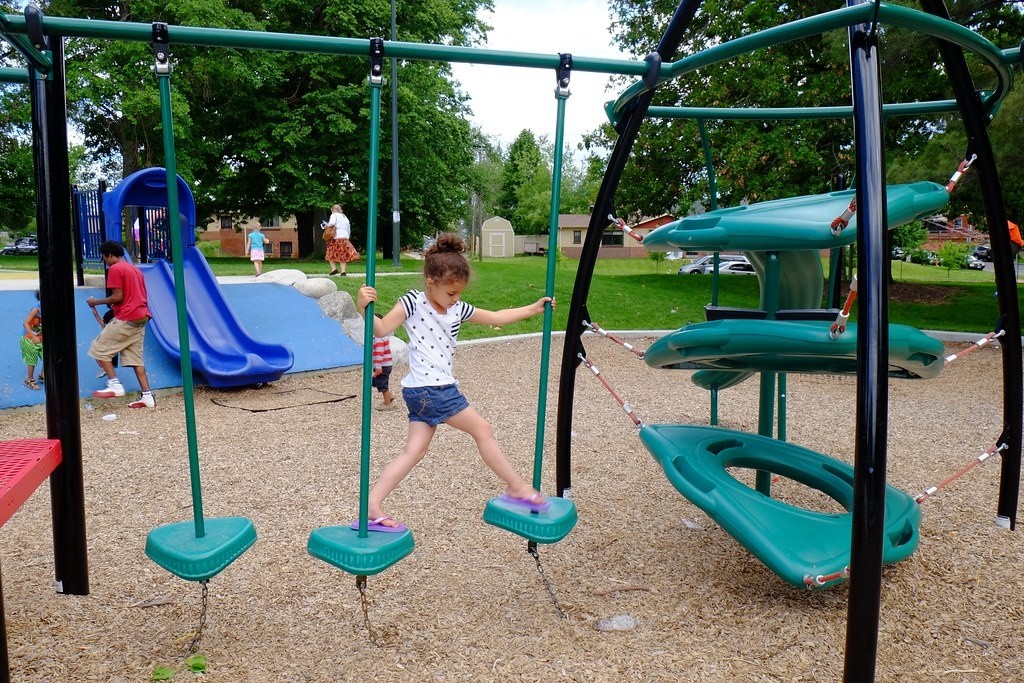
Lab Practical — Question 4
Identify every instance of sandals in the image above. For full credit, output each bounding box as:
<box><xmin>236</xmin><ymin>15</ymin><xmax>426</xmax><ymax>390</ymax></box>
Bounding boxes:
<box><xmin>23</xmin><ymin>378</ymin><xmax>40</xmax><ymax>391</ymax></box>
<box><xmin>37</xmin><ymin>375</ymin><xmax>44</xmax><ymax>384</ymax></box>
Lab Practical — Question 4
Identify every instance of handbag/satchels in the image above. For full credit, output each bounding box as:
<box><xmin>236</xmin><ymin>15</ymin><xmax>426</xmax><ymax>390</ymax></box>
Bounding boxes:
<box><xmin>322</xmin><ymin>225</ymin><xmax>337</xmax><ymax>242</ymax></box>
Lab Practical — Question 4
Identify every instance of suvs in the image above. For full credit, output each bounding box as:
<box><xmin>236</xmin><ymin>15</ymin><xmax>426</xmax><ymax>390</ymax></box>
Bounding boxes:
<box><xmin>678</xmin><ymin>254</ymin><xmax>750</xmax><ymax>275</ymax></box>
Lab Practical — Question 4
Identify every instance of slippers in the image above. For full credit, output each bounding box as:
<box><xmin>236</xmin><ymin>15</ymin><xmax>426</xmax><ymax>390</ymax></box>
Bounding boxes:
<box><xmin>500</xmin><ymin>487</ymin><xmax>551</xmax><ymax>511</ymax></box>
<box><xmin>350</xmin><ymin>514</ymin><xmax>407</xmax><ymax>533</ymax></box>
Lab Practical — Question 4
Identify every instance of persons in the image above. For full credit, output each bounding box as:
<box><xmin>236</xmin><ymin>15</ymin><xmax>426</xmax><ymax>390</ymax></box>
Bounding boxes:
<box><xmin>21</xmin><ymin>288</ymin><xmax>44</xmax><ymax>390</ymax></box>
<box><xmin>926</xmin><ymin>251</ymin><xmax>937</xmax><ymax>261</ymax></box>
<box><xmin>86</xmin><ymin>242</ymin><xmax>161</xmax><ymax>409</ymax></box>
<box><xmin>132</xmin><ymin>207</ymin><xmax>166</xmax><ymax>262</ymax></box>
<box><xmin>244</xmin><ymin>219</ymin><xmax>271</xmax><ymax>278</ymax></box>
<box><xmin>354</xmin><ymin>234</ymin><xmax>556</xmax><ymax>530</ymax></box>
<box><xmin>372</xmin><ymin>314</ymin><xmax>399</xmax><ymax>411</ymax></box>
<box><xmin>322</xmin><ymin>204</ymin><xmax>360</xmax><ymax>278</ymax></box>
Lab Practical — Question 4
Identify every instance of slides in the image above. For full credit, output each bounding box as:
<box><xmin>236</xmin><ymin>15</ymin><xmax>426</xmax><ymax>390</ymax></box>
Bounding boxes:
<box><xmin>116</xmin><ymin>247</ymin><xmax>293</xmax><ymax>387</ymax></box>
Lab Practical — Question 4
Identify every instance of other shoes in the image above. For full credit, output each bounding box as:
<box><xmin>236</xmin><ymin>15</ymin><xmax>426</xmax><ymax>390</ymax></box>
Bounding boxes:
<box><xmin>341</xmin><ymin>272</ymin><xmax>347</xmax><ymax>276</ymax></box>
<box><xmin>329</xmin><ymin>269</ymin><xmax>339</xmax><ymax>275</ymax></box>
<box><xmin>375</xmin><ymin>403</ymin><xmax>394</xmax><ymax>411</ymax></box>
<box><xmin>379</xmin><ymin>398</ymin><xmax>397</xmax><ymax>407</ymax></box>
<box><xmin>256</xmin><ymin>271</ymin><xmax>262</xmax><ymax>277</ymax></box>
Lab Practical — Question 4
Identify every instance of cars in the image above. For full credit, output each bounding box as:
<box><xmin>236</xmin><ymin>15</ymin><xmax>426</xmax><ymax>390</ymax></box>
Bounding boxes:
<box><xmin>891</xmin><ymin>243</ymin><xmax>993</xmax><ymax>271</ymax></box>
<box><xmin>705</xmin><ymin>260</ymin><xmax>758</xmax><ymax>275</ymax></box>
<box><xmin>3</xmin><ymin>234</ymin><xmax>38</xmax><ymax>257</ymax></box>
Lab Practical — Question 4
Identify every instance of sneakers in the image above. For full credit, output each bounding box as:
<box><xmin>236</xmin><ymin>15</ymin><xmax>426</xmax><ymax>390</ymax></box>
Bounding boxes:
<box><xmin>126</xmin><ymin>390</ymin><xmax>157</xmax><ymax>408</ymax></box>
<box><xmin>93</xmin><ymin>378</ymin><xmax>126</xmax><ymax>398</ymax></box>
<box><xmin>97</xmin><ymin>368</ymin><xmax>107</xmax><ymax>378</ymax></box>
